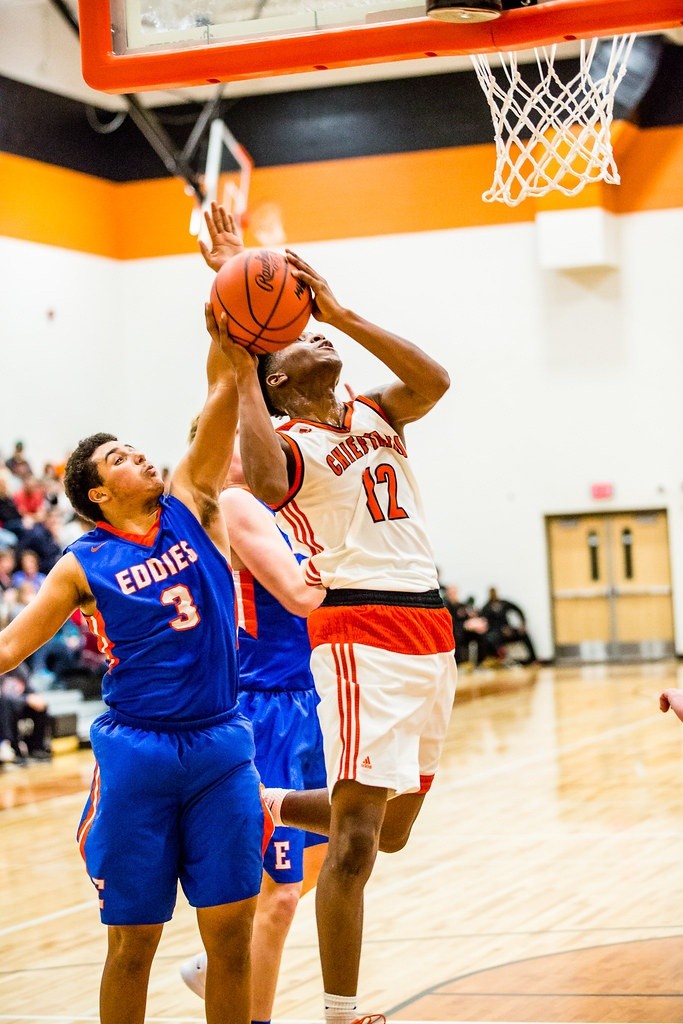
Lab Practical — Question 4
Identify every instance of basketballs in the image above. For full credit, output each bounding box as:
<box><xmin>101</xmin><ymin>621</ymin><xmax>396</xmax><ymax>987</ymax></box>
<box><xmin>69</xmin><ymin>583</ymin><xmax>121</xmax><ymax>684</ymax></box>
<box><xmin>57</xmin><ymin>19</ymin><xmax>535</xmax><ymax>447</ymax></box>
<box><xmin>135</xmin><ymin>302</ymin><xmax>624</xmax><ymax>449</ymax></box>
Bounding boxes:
<box><xmin>211</xmin><ymin>250</ymin><xmax>313</xmax><ymax>353</ymax></box>
